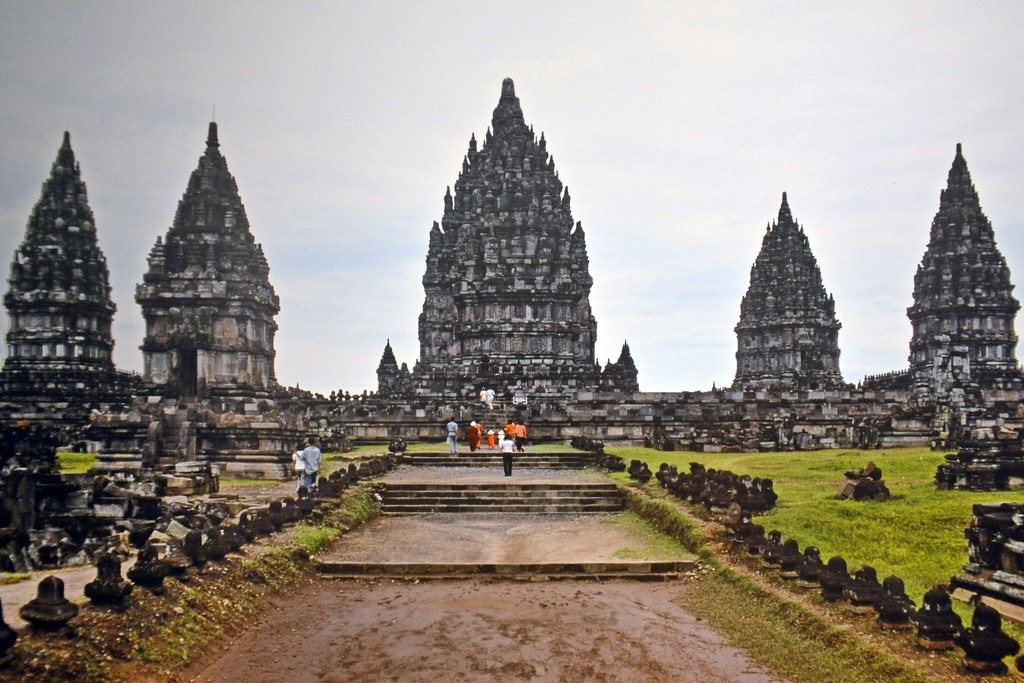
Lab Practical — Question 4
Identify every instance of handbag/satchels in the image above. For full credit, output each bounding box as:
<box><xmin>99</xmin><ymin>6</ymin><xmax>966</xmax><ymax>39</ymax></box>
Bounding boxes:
<box><xmin>446</xmin><ymin>437</ymin><xmax>450</xmax><ymax>444</ymax></box>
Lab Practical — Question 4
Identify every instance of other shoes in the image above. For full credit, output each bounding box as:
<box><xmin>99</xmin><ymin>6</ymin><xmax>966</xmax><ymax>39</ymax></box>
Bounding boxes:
<box><xmin>518</xmin><ymin>447</ymin><xmax>524</xmax><ymax>453</ymax></box>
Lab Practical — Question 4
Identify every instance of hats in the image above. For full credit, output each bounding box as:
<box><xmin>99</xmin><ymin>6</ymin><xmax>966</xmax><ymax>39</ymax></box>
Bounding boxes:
<box><xmin>481</xmin><ymin>387</ymin><xmax>485</xmax><ymax>390</ymax></box>
<box><xmin>488</xmin><ymin>430</ymin><xmax>494</xmax><ymax>435</ymax></box>
<box><xmin>498</xmin><ymin>430</ymin><xmax>505</xmax><ymax>435</ymax></box>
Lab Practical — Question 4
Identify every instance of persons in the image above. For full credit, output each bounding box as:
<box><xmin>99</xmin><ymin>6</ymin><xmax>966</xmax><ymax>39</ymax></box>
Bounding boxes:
<box><xmin>292</xmin><ymin>437</ymin><xmax>321</xmax><ymax>499</ymax></box>
<box><xmin>465</xmin><ymin>419</ymin><xmax>527</xmax><ymax>452</ymax></box>
<box><xmin>500</xmin><ymin>436</ymin><xmax>514</xmax><ymax>476</ymax></box>
<box><xmin>479</xmin><ymin>384</ymin><xmax>497</xmax><ymax>411</ymax></box>
<box><xmin>444</xmin><ymin>416</ymin><xmax>459</xmax><ymax>453</ymax></box>
